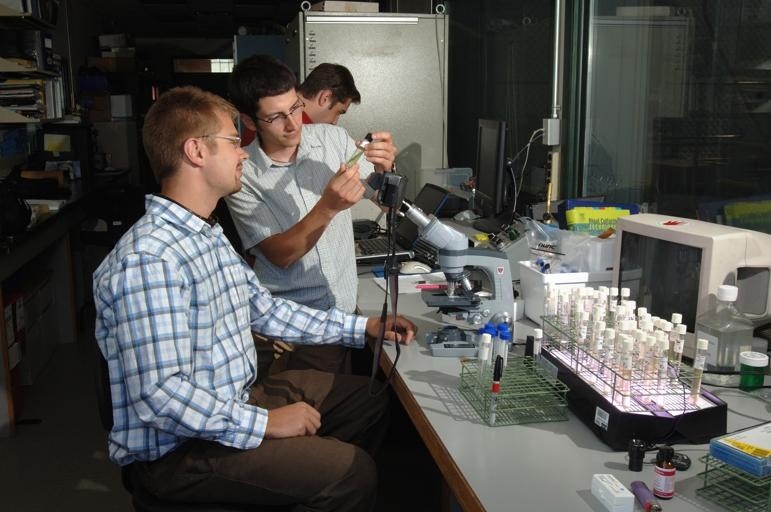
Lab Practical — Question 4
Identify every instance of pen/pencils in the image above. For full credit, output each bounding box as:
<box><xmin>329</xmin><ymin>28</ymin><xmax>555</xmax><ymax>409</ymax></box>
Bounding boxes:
<box><xmin>489</xmin><ymin>355</ymin><xmax>503</xmax><ymax>426</ymax></box>
<box><xmin>415</xmin><ymin>280</ymin><xmax>448</xmax><ymax>289</ymax></box>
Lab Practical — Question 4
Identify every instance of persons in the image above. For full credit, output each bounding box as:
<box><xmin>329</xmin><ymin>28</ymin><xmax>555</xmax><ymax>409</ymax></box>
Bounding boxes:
<box><xmin>223</xmin><ymin>56</ymin><xmax>400</xmax><ymax>380</ymax></box>
<box><xmin>234</xmin><ymin>62</ymin><xmax>363</xmax><ymax>151</ymax></box>
<box><xmin>91</xmin><ymin>84</ymin><xmax>421</xmax><ymax>512</ymax></box>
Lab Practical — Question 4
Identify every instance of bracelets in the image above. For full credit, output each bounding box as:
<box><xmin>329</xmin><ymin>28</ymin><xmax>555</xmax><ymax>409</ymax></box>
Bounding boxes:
<box><xmin>372</xmin><ymin>162</ymin><xmax>398</xmax><ymax>174</ymax></box>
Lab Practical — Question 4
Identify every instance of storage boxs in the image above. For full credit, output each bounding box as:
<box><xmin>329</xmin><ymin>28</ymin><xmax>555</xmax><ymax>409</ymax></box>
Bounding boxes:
<box><xmin>87</xmin><ymin>55</ymin><xmax>137</xmax><ymax>74</ymax></box>
<box><xmin>517</xmin><ymin>260</ymin><xmax>590</xmax><ymax>327</ymax></box>
<box><xmin>2</xmin><ymin>272</ymin><xmax>59</xmax><ymax>386</ymax></box>
<box><xmin>79</xmin><ymin>95</ymin><xmax>134</xmax><ymax>121</ymax></box>
<box><xmin>621</xmin><ymin>268</ymin><xmax>644</xmax><ymax>300</ymax></box>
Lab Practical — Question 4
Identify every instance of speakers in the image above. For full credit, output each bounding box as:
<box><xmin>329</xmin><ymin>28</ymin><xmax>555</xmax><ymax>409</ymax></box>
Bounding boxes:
<box><xmin>731</xmin><ymin>264</ymin><xmax>771</xmax><ymax>329</ymax></box>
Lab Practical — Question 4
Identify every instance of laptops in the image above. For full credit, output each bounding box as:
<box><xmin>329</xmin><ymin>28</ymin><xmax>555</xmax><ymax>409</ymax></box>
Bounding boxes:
<box><xmin>353</xmin><ymin>181</ymin><xmax>449</xmax><ymax>264</ymax></box>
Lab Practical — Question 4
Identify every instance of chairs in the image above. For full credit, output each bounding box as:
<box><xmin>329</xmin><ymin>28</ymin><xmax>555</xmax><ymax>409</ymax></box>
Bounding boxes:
<box><xmin>93</xmin><ymin>353</ymin><xmax>296</xmax><ymax>512</ymax></box>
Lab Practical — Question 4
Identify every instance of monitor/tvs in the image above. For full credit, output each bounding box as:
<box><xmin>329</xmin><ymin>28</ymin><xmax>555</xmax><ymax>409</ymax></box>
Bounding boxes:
<box><xmin>610</xmin><ymin>210</ymin><xmax>771</xmax><ymax>362</ymax></box>
<box><xmin>473</xmin><ymin>116</ymin><xmax>508</xmax><ymax>214</ymax></box>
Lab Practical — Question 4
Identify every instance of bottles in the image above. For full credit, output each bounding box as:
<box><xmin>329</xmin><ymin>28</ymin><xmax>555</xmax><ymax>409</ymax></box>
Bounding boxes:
<box><xmin>655</xmin><ymin>447</ymin><xmax>676</xmax><ymax>499</ymax></box>
<box><xmin>344</xmin><ymin>132</ymin><xmax>373</xmax><ymax>168</ymax></box>
<box><xmin>696</xmin><ymin>286</ymin><xmax>769</xmax><ymax>391</ymax></box>
<box><xmin>544</xmin><ymin>286</ymin><xmax>707</xmax><ymax>407</ymax></box>
<box><xmin>477</xmin><ymin>323</ymin><xmax>543</xmax><ymax>372</ymax></box>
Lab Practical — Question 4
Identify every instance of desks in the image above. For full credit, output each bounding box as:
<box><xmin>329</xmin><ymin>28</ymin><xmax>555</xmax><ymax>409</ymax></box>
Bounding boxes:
<box><xmin>0</xmin><ymin>166</ymin><xmax>133</xmax><ymax>439</ymax></box>
<box><xmin>352</xmin><ymin>265</ymin><xmax>771</xmax><ymax>512</ymax></box>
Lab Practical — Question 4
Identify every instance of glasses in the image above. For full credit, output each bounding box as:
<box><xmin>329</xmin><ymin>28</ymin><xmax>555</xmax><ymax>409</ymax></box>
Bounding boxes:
<box><xmin>248</xmin><ymin>95</ymin><xmax>306</xmax><ymax>127</ymax></box>
<box><xmin>180</xmin><ymin>135</ymin><xmax>242</xmax><ymax>152</ymax></box>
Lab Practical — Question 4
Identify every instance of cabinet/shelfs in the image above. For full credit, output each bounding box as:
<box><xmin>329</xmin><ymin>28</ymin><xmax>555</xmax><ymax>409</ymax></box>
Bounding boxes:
<box><xmin>0</xmin><ymin>0</ymin><xmax>68</xmax><ymax>125</ymax></box>
